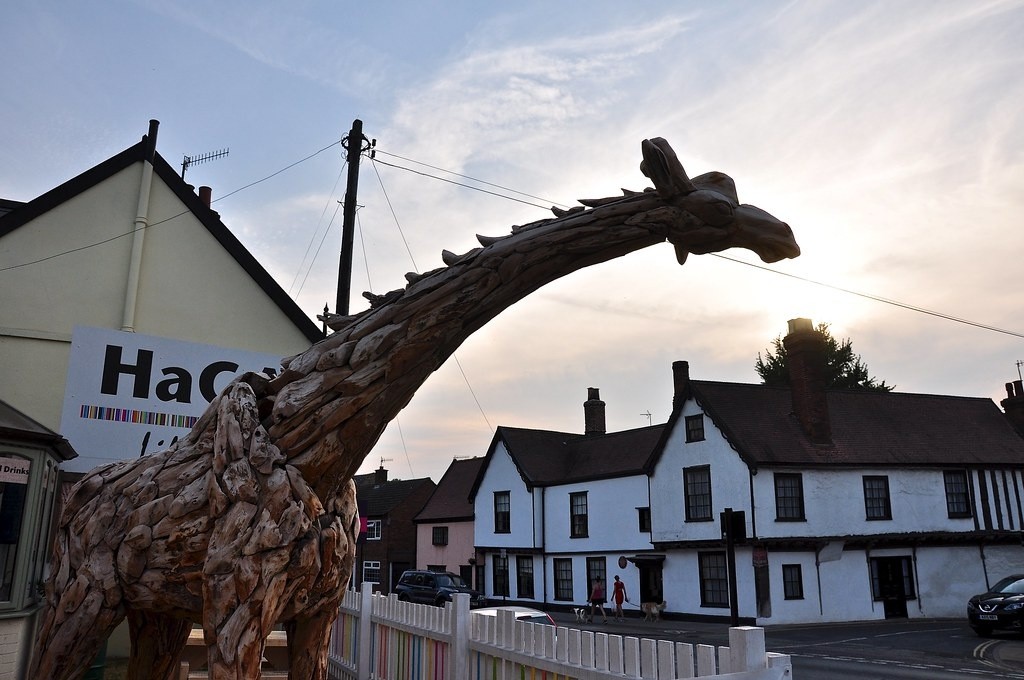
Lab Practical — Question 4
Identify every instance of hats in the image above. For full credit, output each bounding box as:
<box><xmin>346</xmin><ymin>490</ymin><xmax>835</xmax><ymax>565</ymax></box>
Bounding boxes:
<box><xmin>595</xmin><ymin>576</ymin><xmax>602</xmax><ymax>580</ymax></box>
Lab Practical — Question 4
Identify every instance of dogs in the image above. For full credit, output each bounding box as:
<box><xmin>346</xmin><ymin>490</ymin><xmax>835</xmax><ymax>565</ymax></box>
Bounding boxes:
<box><xmin>573</xmin><ymin>608</ymin><xmax>593</xmax><ymax>626</ymax></box>
<box><xmin>640</xmin><ymin>601</ymin><xmax>666</xmax><ymax>624</ymax></box>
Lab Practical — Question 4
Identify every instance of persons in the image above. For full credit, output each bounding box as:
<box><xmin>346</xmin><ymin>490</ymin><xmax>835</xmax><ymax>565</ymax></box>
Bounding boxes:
<box><xmin>588</xmin><ymin>576</ymin><xmax>607</xmax><ymax>625</ymax></box>
<box><xmin>611</xmin><ymin>575</ymin><xmax>628</xmax><ymax>621</ymax></box>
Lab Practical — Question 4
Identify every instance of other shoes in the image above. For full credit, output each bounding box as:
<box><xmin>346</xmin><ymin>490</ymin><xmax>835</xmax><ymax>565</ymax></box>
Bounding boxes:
<box><xmin>602</xmin><ymin>620</ymin><xmax>608</xmax><ymax>624</ymax></box>
<box><xmin>620</xmin><ymin>619</ymin><xmax>625</xmax><ymax>623</ymax></box>
<box><xmin>613</xmin><ymin>618</ymin><xmax>619</xmax><ymax>622</ymax></box>
<box><xmin>588</xmin><ymin>619</ymin><xmax>592</xmax><ymax>624</ymax></box>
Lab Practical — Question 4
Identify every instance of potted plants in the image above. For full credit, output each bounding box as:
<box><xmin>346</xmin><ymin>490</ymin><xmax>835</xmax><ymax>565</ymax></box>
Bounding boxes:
<box><xmin>469</xmin><ymin>557</ymin><xmax>475</xmax><ymax>565</ymax></box>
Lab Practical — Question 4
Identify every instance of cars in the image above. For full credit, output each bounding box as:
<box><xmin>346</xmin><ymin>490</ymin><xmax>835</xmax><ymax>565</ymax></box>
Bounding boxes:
<box><xmin>468</xmin><ymin>606</ymin><xmax>558</xmax><ymax>646</ymax></box>
<box><xmin>967</xmin><ymin>574</ymin><xmax>1024</xmax><ymax>637</ymax></box>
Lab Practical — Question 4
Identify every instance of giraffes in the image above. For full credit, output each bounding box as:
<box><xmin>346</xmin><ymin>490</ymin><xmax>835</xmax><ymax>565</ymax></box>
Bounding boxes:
<box><xmin>23</xmin><ymin>137</ymin><xmax>801</xmax><ymax>679</ymax></box>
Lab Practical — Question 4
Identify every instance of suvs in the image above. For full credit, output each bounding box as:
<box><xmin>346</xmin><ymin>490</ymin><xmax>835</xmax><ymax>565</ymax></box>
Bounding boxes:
<box><xmin>395</xmin><ymin>570</ymin><xmax>489</xmax><ymax>611</ymax></box>
